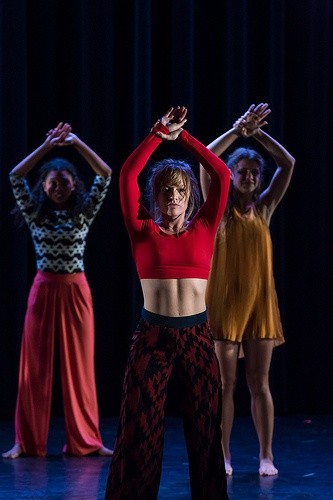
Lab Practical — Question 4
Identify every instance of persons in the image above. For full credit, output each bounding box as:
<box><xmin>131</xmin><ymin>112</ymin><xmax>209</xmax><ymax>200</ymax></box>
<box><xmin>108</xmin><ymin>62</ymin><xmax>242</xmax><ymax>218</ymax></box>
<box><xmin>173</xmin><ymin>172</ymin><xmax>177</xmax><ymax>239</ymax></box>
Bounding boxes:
<box><xmin>105</xmin><ymin>105</ymin><xmax>230</xmax><ymax>500</ymax></box>
<box><xmin>3</xmin><ymin>122</ymin><xmax>114</xmax><ymax>459</ymax></box>
<box><xmin>200</xmin><ymin>103</ymin><xmax>295</xmax><ymax>477</ymax></box>
